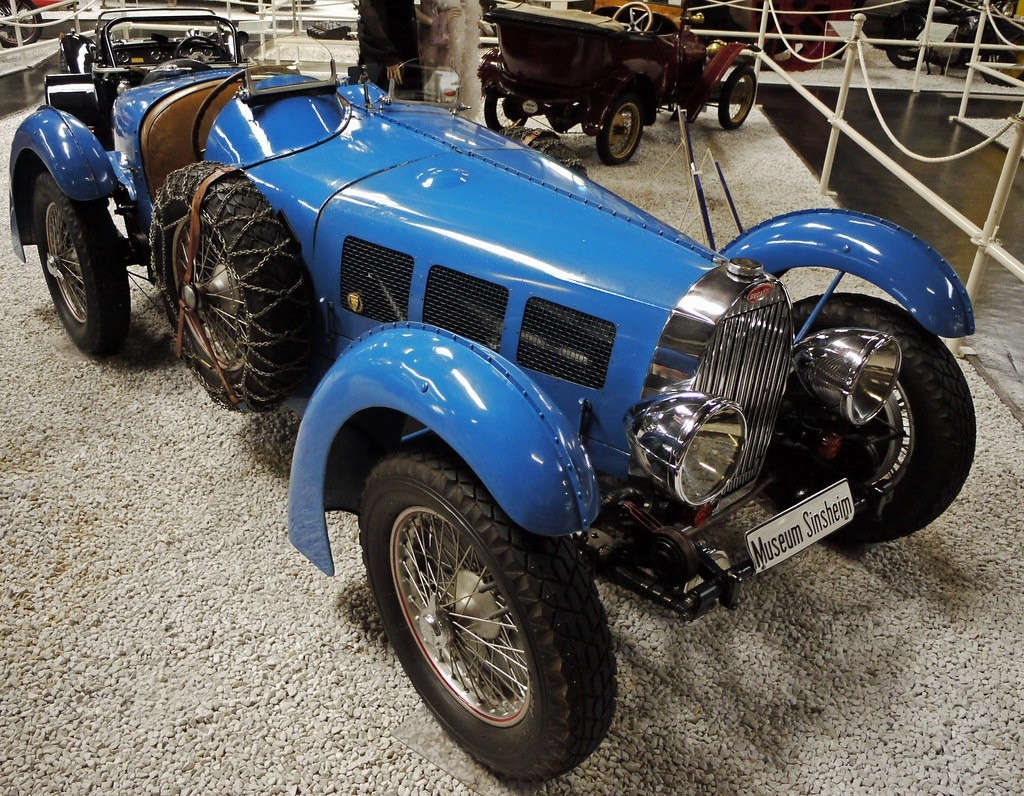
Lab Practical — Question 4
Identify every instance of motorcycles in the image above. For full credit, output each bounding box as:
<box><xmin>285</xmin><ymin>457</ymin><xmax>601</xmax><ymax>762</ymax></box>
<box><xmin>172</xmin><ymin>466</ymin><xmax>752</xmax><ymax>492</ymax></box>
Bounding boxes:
<box><xmin>863</xmin><ymin>1</ymin><xmax>1024</xmax><ymax>86</ymax></box>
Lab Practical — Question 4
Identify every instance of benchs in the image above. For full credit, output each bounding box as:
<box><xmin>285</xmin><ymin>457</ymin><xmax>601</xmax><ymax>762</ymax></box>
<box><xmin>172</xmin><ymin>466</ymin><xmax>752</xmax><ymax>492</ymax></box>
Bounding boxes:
<box><xmin>501</xmin><ymin>3</ymin><xmax>624</xmax><ymax>32</ymax></box>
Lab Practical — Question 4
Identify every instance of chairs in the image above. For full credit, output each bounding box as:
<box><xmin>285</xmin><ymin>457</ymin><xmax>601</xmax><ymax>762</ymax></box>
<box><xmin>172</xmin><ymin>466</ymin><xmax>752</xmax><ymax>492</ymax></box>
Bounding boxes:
<box><xmin>139</xmin><ymin>74</ymin><xmax>270</xmax><ymax>216</ymax></box>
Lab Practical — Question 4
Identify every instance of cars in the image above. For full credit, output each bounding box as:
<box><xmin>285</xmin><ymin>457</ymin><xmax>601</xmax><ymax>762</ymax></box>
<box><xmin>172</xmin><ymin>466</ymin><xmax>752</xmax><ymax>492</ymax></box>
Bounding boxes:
<box><xmin>478</xmin><ymin>3</ymin><xmax>760</xmax><ymax>167</ymax></box>
<box><xmin>8</xmin><ymin>5</ymin><xmax>979</xmax><ymax>781</ymax></box>
